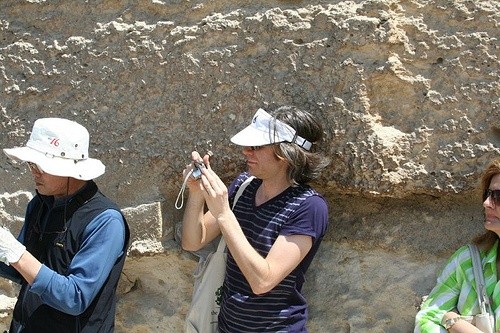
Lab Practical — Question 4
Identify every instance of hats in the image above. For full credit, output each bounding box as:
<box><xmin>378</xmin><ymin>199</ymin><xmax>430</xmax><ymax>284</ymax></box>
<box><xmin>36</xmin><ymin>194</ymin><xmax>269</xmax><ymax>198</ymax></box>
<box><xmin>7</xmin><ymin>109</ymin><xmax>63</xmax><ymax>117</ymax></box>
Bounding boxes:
<box><xmin>3</xmin><ymin>117</ymin><xmax>106</xmax><ymax>182</ymax></box>
<box><xmin>229</xmin><ymin>107</ymin><xmax>314</xmax><ymax>151</ymax></box>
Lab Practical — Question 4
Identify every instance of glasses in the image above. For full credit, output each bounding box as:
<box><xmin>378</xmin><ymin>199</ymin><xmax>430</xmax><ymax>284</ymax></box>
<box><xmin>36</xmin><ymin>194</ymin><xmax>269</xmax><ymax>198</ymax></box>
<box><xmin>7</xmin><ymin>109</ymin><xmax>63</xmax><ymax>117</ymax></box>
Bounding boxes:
<box><xmin>482</xmin><ymin>188</ymin><xmax>500</xmax><ymax>205</ymax></box>
<box><xmin>250</xmin><ymin>141</ymin><xmax>286</xmax><ymax>151</ymax></box>
<box><xmin>27</xmin><ymin>161</ymin><xmax>45</xmax><ymax>174</ymax></box>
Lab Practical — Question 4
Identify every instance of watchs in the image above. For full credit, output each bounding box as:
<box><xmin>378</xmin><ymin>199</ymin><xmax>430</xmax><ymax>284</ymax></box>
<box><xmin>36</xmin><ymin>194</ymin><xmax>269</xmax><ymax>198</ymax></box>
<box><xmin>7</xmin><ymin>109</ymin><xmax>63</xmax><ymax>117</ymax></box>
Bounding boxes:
<box><xmin>443</xmin><ymin>316</ymin><xmax>463</xmax><ymax>330</ymax></box>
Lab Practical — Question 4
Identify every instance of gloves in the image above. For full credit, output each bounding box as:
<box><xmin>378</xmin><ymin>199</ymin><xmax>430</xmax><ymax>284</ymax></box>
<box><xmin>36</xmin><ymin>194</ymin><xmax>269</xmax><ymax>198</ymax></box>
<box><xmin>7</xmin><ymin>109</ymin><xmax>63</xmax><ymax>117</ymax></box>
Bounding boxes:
<box><xmin>0</xmin><ymin>227</ymin><xmax>27</xmax><ymax>266</ymax></box>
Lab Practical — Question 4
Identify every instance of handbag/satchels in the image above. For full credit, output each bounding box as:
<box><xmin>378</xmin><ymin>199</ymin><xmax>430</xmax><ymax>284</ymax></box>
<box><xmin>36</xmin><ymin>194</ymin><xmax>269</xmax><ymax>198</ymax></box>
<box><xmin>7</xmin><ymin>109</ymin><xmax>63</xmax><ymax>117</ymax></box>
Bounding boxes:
<box><xmin>182</xmin><ymin>175</ymin><xmax>256</xmax><ymax>332</ymax></box>
<box><xmin>456</xmin><ymin>243</ymin><xmax>496</xmax><ymax>333</ymax></box>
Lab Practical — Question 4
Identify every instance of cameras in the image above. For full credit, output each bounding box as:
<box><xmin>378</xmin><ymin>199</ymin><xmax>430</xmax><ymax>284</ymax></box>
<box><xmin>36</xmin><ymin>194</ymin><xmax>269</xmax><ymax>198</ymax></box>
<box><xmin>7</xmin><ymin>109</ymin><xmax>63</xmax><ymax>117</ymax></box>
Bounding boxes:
<box><xmin>191</xmin><ymin>161</ymin><xmax>207</xmax><ymax>181</ymax></box>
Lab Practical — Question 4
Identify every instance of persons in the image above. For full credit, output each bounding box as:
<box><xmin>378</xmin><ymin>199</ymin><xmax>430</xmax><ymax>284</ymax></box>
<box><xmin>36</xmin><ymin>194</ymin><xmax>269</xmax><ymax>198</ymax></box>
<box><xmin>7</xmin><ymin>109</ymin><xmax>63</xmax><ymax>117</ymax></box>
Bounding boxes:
<box><xmin>0</xmin><ymin>118</ymin><xmax>130</xmax><ymax>333</ymax></box>
<box><xmin>413</xmin><ymin>157</ymin><xmax>500</xmax><ymax>333</ymax></box>
<box><xmin>179</xmin><ymin>105</ymin><xmax>329</xmax><ymax>332</ymax></box>
